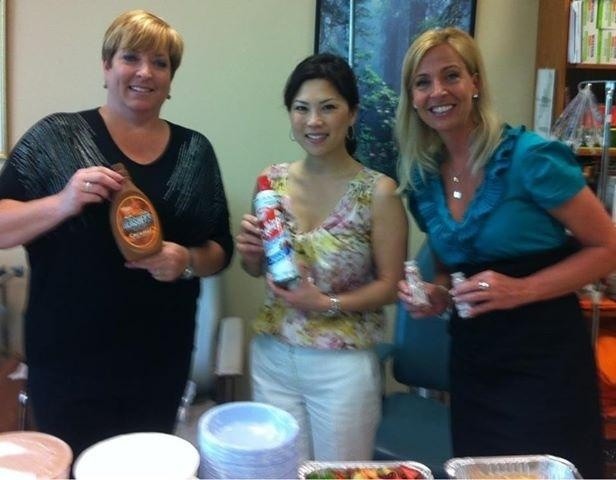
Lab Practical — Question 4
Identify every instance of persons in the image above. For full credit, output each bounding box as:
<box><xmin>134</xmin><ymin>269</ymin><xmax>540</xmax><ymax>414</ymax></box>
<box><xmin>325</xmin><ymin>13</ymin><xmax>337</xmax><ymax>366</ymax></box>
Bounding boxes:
<box><xmin>399</xmin><ymin>25</ymin><xmax>615</xmax><ymax>479</ymax></box>
<box><xmin>0</xmin><ymin>6</ymin><xmax>233</xmax><ymax>478</ymax></box>
<box><xmin>238</xmin><ymin>50</ymin><xmax>408</xmax><ymax>470</ymax></box>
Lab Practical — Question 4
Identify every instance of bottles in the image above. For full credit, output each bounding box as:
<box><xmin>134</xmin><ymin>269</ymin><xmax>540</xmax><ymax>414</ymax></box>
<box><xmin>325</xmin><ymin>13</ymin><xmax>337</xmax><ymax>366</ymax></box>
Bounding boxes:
<box><xmin>450</xmin><ymin>272</ymin><xmax>476</xmax><ymax>319</ymax></box>
<box><xmin>108</xmin><ymin>159</ymin><xmax>162</xmax><ymax>263</ymax></box>
<box><xmin>402</xmin><ymin>259</ymin><xmax>429</xmax><ymax>306</ymax></box>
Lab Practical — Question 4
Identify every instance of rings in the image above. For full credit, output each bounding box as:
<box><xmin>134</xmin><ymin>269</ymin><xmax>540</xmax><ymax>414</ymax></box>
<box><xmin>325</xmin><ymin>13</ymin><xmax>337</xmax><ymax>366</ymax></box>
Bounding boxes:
<box><xmin>83</xmin><ymin>181</ymin><xmax>91</xmax><ymax>193</ymax></box>
<box><xmin>478</xmin><ymin>281</ymin><xmax>490</xmax><ymax>290</ymax></box>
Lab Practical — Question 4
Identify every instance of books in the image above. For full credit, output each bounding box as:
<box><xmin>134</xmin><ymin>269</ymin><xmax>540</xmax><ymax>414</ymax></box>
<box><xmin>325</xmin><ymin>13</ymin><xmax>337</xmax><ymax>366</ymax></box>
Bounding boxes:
<box><xmin>565</xmin><ymin>0</ymin><xmax>616</xmax><ymax>66</ymax></box>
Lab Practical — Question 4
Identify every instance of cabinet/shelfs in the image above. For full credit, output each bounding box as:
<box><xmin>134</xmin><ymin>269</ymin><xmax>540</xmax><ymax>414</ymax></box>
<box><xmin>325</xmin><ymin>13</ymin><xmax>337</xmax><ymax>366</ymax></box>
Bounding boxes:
<box><xmin>529</xmin><ymin>0</ymin><xmax>616</xmax><ymax>319</ymax></box>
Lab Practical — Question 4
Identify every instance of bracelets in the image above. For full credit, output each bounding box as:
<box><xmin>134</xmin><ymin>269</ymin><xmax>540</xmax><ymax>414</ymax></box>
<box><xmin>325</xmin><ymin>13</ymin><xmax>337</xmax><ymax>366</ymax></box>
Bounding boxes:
<box><xmin>181</xmin><ymin>246</ymin><xmax>196</xmax><ymax>281</ymax></box>
<box><xmin>320</xmin><ymin>292</ymin><xmax>342</xmax><ymax>320</ymax></box>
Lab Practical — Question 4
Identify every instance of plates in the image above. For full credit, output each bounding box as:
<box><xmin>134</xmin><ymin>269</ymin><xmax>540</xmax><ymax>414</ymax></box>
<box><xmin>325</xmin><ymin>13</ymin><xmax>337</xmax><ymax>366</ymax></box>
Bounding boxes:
<box><xmin>198</xmin><ymin>400</ymin><xmax>300</xmax><ymax>478</ymax></box>
<box><xmin>74</xmin><ymin>432</ymin><xmax>200</xmax><ymax>479</ymax></box>
<box><xmin>0</xmin><ymin>431</ymin><xmax>72</xmax><ymax>480</ymax></box>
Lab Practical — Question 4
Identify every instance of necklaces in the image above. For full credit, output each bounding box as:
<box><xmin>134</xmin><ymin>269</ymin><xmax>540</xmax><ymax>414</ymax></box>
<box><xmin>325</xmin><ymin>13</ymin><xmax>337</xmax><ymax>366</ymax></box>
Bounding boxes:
<box><xmin>447</xmin><ymin>160</ymin><xmax>469</xmax><ymax>201</ymax></box>
